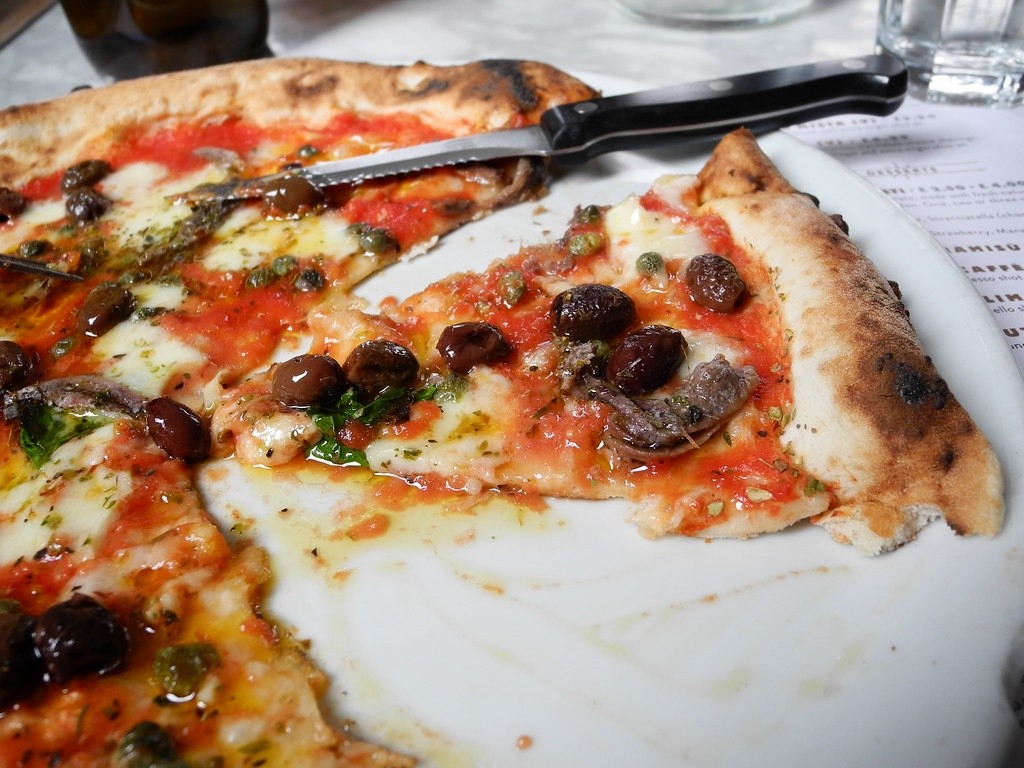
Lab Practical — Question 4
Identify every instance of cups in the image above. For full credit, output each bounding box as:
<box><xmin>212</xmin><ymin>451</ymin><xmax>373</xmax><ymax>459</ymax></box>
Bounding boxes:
<box><xmin>870</xmin><ymin>0</ymin><xmax>1024</xmax><ymax>107</ymax></box>
<box><xmin>59</xmin><ymin>0</ymin><xmax>268</xmax><ymax>78</ymax></box>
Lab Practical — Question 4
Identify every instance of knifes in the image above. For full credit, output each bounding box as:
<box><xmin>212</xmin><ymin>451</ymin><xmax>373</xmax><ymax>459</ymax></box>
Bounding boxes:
<box><xmin>157</xmin><ymin>51</ymin><xmax>907</xmax><ymax>207</ymax></box>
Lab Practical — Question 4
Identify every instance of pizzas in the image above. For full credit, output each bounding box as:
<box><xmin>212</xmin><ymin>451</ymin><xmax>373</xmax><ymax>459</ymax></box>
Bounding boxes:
<box><xmin>0</xmin><ymin>54</ymin><xmax>1008</xmax><ymax>768</ymax></box>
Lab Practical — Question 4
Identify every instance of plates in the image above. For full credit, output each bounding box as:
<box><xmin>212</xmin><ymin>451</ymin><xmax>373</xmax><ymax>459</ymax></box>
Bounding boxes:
<box><xmin>0</xmin><ymin>71</ymin><xmax>1022</xmax><ymax>768</ymax></box>
